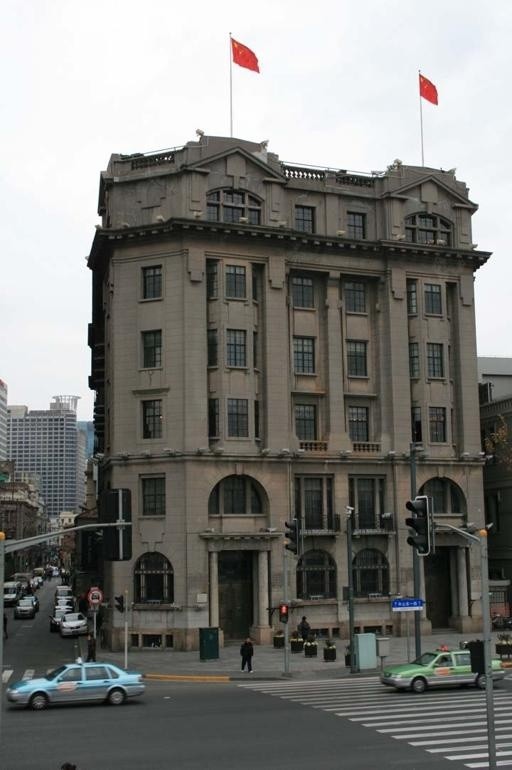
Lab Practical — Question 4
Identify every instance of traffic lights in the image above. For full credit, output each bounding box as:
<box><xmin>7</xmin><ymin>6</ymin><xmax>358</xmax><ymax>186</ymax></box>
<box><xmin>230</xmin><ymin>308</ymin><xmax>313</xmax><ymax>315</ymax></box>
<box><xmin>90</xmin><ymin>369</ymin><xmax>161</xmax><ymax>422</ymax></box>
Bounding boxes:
<box><xmin>115</xmin><ymin>595</ymin><xmax>124</xmax><ymax>612</ymax></box>
<box><xmin>405</xmin><ymin>495</ymin><xmax>431</xmax><ymax>556</ymax></box>
<box><xmin>279</xmin><ymin>603</ymin><xmax>289</xmax><ymax>623</ymax></box>
<box><xmin>285</xmin><ymin>521</ymin><xmax>300</xmax><ymax>555</ymax></box>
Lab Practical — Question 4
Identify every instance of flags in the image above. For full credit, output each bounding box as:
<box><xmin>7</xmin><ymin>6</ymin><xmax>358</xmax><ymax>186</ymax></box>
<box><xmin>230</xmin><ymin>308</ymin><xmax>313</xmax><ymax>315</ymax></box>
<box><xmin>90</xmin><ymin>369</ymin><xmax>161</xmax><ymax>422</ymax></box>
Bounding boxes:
<box><xmin>232</xmin><ymin>38</ymin><xmax>260</xmax><ymax>74</ymax></box>
<box><xmin>420</xmin><ymin>74</ymin><xmax>438</xmax><ymax>106</ymax></box>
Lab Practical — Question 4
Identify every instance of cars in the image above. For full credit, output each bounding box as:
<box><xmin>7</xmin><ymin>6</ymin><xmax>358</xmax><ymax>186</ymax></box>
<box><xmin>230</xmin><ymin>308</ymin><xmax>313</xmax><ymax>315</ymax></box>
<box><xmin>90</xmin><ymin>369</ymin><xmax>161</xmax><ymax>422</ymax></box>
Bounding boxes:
<box><xmin>51</xmin><ymin>586</ymin><xmax>89</xmax><ymax>638</ymax></box>
<box><xmin>6</xmin><ymin>656</ymin><xmax>146</xmax><ymax>710</ymax></box>
<box><xmin>4</xmin><ymin>566</ymin><xmax>59</xmax><ymax>618</ymax></box>
<box><xmin>380</xmin><ymin>646</ymin><xmax>506</xmax><ymax>694</ymax></box>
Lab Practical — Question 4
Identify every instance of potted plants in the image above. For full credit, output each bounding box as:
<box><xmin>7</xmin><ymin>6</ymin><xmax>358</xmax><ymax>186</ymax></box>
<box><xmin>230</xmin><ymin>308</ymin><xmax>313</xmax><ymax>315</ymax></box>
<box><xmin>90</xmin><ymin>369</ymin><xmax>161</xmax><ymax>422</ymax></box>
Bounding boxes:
<box><xmin>273</xmin><ymin>631</ymin><xmax>356</xmax><ymax>667</ymax></box>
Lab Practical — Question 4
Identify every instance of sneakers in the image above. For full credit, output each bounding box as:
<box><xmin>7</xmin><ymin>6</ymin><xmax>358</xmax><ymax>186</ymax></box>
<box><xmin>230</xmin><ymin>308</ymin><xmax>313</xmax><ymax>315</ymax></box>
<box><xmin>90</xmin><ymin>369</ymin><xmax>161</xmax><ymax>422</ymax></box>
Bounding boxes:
<box><xmin>240</xmin><ymin>670</ymin><xmax>254</xmax><ymax>674</ymax></box>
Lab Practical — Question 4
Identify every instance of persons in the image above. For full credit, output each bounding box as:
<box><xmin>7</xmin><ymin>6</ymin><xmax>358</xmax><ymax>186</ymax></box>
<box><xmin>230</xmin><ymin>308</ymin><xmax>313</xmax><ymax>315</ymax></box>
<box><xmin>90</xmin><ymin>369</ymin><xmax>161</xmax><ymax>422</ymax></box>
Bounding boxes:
<box><xmin>297</xmin><ymin>616</ymin><xmax>311</xmax><ymax>640</ymax></box>
<box><xmin>240</xmin><ymin>635</ymin><xmax>254</xmax><ymax>673</ymax></box>
<box><xmin>86</xmin><ymin>633</ymin><xmax>97</xmax><ymax>662</ymax></box>
